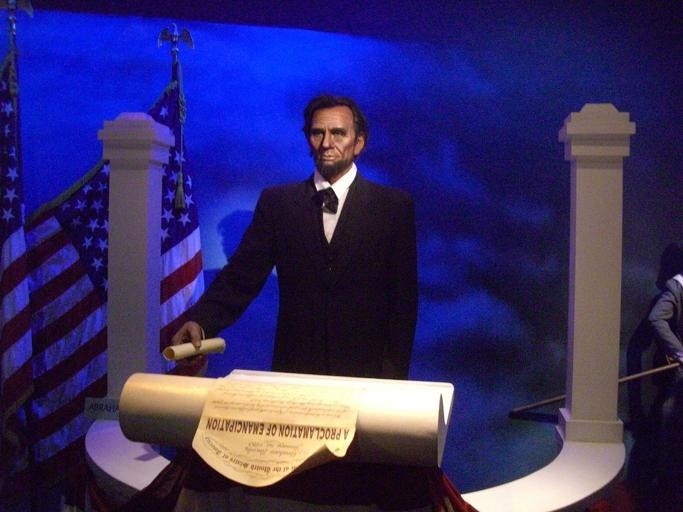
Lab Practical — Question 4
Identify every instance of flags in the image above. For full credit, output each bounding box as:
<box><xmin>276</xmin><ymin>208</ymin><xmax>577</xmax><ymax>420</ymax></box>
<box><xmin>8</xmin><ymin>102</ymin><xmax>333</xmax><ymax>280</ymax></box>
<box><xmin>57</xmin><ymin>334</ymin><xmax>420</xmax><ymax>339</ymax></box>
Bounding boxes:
<box><xmin>25</xmin><ymin>82</ymin><xmax>208</xmax><ymax>491</ymax></box>
<box><xmin>0</xmin><ymin>54</ymin><xmax>32</xmax><ymax>511</ymax></box>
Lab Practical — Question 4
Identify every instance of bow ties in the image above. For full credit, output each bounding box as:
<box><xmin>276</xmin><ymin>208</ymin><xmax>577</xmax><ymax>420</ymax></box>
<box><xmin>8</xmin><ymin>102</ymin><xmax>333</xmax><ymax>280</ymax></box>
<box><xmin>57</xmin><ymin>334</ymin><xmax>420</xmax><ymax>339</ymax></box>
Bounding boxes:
<box><xmin>312</xmin><ymin>187</ymin><xmax>338</xmax><ymax>213</ymax></box>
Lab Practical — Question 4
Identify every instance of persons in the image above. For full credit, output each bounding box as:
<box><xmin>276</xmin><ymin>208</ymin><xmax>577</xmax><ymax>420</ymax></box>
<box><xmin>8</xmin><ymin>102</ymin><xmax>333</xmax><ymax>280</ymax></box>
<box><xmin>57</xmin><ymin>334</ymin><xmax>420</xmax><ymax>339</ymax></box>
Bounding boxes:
<box><xmin>169</xmin><ymin>94</ymin><xmax>418</xmax><ymax>380</ymax></box>
<box><xmin>648</xmin><ymin>273</ymin><xmax>682</xmax><ymax>388</ymax></box>
